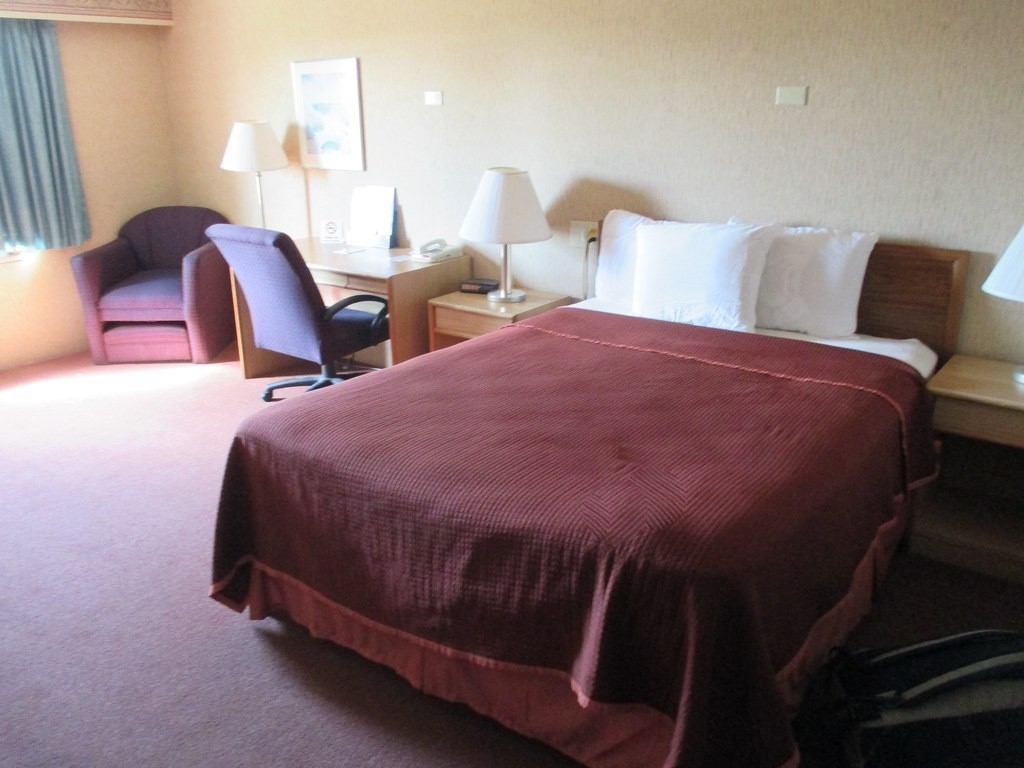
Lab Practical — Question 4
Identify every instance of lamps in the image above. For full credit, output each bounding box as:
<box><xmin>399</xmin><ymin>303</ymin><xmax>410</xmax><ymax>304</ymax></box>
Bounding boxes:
<box><xmin>219</xmin><ymin>120</ymin><xmax>290</xmax><ymax>229</ymax></box>
<box><xmin>459</xmin><ymin>167</ymin><xmax>555</xmax><ymax>303</ymax></box>
<box><xmin>981</xmin><ymin>225</ymin><xmax>1024</xmax><ymax>385</ymax></box>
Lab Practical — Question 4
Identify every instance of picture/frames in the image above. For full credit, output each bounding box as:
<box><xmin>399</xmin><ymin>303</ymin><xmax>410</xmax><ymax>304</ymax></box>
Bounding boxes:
<box><xmin>290</xmin><ymin>57</ymin><xmax>366</xmax><ymax>173</ymax></box>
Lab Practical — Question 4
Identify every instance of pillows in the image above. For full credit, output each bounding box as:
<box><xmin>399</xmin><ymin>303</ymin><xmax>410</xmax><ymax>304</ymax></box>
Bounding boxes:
<box><xmin>725</xmin><ymin>215</ymin><xmax>881</xmax><ymax>338</ymax></box>
<box><xmin>593</xmin><ymin>209</ymin><xmax>779</xmax><ymax>333</ymax></box>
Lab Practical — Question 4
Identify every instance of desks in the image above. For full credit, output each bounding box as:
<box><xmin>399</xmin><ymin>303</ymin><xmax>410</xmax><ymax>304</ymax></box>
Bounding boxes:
<box><xmin>230</xmin><ymin>247</ymin><xmax>473</xmax><ymax>381</ymax></box>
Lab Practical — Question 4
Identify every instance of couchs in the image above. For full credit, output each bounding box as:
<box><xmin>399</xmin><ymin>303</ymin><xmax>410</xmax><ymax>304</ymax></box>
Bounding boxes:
<box><xmin>69</xmin><ymin>205</ymin><xmax>236</xmax><ymax>365</ymax></box>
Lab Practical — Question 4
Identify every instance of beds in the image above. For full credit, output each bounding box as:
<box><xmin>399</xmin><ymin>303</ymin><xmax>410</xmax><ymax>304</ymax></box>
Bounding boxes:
<box><xmin>232</xmin><ymin>297</ymin><xmax>941</xmax><ymax>760</ymax></box>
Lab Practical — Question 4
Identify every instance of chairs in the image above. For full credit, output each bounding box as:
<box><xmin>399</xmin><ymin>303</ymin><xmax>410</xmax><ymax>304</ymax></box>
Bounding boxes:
<box><xmin>204</xmin><ymin>223</ymin><xmax>390</xmax><ymax>403</ymax></box>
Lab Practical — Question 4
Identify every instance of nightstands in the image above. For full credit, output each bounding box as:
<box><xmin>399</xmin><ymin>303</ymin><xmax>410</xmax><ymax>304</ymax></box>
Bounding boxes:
<box><xmin>908</xmin><ymin>354</ymin><xmax>1024</xmax><ymax>588</ymax></box>
<box><xmin>427</xmin><ymin>287</ymin><xmax>573</xmax><ymax>356</ymax></box>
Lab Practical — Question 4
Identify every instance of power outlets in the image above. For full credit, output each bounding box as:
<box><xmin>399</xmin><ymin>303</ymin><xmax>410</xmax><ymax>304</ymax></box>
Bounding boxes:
<box><xmin>568</xmin><ymin>221</ymin><xmax>600</xmax><ymax>249</ymax></box>
